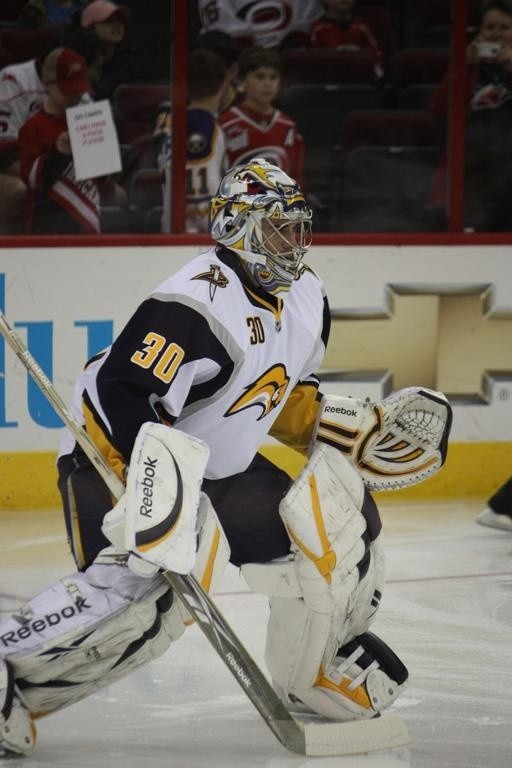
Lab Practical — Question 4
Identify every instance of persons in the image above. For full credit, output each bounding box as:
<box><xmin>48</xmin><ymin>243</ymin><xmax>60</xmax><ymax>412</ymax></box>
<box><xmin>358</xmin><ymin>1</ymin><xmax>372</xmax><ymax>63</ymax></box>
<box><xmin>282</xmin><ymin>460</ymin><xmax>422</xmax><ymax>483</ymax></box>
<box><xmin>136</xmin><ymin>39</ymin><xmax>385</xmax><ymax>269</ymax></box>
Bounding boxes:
<box><xmin>429</xmin><ymin>0</ymin><xmax>512</xmax><ymax>231</ymax></box>
<box><xmin>0</xmin><ymin>158</ymin><xmax>453</xmax><ymax>757</ymax></box>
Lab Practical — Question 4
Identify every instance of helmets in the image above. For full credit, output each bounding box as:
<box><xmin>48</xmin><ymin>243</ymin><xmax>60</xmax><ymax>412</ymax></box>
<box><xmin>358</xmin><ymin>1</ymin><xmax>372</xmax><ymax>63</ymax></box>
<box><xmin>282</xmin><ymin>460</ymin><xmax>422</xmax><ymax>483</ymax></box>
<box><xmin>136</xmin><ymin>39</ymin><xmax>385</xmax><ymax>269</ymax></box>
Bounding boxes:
<box><xmin>210</xmin><ymin>159</ymin><xmax>313</xmax><ymax>292</ymax></box>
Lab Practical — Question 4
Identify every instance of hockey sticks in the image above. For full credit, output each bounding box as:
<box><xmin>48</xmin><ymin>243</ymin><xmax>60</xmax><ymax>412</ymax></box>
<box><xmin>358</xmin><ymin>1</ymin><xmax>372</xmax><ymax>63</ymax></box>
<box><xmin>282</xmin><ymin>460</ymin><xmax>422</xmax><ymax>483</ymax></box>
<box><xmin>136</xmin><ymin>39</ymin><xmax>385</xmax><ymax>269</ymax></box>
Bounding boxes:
<box><xmin>0</xmin><ymin>313</ymin><xmax>409</xmax><ymax>757</ymax></box>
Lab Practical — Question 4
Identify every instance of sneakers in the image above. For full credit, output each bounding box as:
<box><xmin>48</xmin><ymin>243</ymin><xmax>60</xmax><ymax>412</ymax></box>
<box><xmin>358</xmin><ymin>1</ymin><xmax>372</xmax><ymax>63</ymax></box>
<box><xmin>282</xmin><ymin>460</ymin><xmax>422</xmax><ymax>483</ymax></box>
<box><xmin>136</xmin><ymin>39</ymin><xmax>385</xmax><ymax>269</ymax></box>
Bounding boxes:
<box><xmin>475</xmin><ymin>507</ymin><xmax>512</xmax><ymax>531</ymax></box>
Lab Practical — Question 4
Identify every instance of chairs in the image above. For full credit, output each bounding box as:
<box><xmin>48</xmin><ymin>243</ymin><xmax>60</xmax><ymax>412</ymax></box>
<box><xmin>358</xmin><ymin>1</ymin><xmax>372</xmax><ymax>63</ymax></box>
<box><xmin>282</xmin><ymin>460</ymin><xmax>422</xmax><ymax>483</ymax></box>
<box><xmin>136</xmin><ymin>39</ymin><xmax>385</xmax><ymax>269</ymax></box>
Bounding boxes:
<box><xmin>0</xmin><ymin>0</ymin><xmax>476</xmax><ymax>230</ymax></box>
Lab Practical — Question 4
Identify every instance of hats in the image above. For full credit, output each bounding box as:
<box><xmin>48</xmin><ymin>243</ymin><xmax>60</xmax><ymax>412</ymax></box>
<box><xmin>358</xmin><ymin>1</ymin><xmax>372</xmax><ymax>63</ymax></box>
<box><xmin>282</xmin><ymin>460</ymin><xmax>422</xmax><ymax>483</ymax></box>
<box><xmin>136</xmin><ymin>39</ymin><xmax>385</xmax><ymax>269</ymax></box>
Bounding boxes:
<box><xmin>41</xmin><ymin>46</ymin><xmax>91</xmax><ymax>95</ymax></box>
<box><xmin>81</xmin><ymin>0</ymin><xmax>120</xmax><ymax>27</ymax></box>
